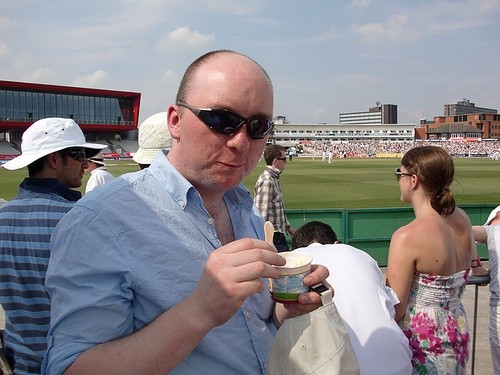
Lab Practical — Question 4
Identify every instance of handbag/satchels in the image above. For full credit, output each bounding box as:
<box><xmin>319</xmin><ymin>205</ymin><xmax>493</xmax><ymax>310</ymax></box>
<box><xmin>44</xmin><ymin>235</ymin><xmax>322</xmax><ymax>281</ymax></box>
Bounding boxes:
<box><xmin>265</xmin><ymin>284</ymin><xmax>362</xmax><ymax>375</ymax></box>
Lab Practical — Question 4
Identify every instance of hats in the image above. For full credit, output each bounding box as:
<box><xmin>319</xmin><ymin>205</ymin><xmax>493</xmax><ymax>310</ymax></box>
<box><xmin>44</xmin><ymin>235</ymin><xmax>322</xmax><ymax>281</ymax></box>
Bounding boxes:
<box><xmin>132</xmin><ymin>111</ymin><xmax>174</xmax><ymax>164</ymax></box>
<box><xmin>1</xmin><ymin>118</ymin><xmax>109</xmax><ymax>171</ymax></box>
<box><xmin>483</xmin><ymin>205</ymin><xmax>500</xmax><ymax>227</ymax></box>
<box><xmin>87</xmin><ymin>151</ymin><xmax>105</xmax><ymax>166</ymax></box>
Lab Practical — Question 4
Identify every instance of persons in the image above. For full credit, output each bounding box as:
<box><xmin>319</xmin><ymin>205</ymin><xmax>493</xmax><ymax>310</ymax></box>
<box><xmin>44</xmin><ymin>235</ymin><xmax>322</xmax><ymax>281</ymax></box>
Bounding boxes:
<box><xmin>472</xmin><ymin>205</ymin><xmax>500</xmax><ymax>375</ymax></box>
<box><xmin>255</xmin><ymin>143</ymin><xmax>297</xmax><ymax>252</ymax></box>
<box><xmin>42</xmin><ymin>50</ymin><xmax>334</xmax><ymax>375</ymax></box>
<box><xmin>0</xmin><ymin>118</ymin><xmax>109</xmax><ymax>375</ymax></box>
<box><xmin>84</xmin><ymin>152</ymin><xmax>114</xmax><ymax>194</ymax></box>
<box><xmin>288</xmin><ymin>140</ymin><xmax>500</xmax><ymax>164</ymax></box>
<box><xmin>386</xmin><ymin>146</ymin><xmax>490</xmax><ymax>375</ymax></box>
<box><xmin>290</xmin><ymin>221</ymin><xmax>413</xmax><ymax>375</ymax></box>
<box><xmin>133</xmin><ymin>111</ymin><xmax>173</xmax><ymax>170</ymax></box>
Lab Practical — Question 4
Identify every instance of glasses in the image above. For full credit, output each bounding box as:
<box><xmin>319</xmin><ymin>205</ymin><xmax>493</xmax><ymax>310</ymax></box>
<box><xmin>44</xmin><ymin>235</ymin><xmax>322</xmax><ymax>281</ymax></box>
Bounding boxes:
<box><xmin>394</xmin><ymin>168</ymin><xmax>414</xmax><ymax>179</ymax></box>
<box><xmin>273</xmin><ymin>157</ymin><xmax>286</xmax><ymax>162</ymax></box>
<box><xmin>56</xmin><ymin>151</ymin><xmax>86</xmax><ymax>162</ymax></box>
<box><xmin>176</xmin><ymin>100</ymin><xmax>275</xmax><ymax>140</ymax></box>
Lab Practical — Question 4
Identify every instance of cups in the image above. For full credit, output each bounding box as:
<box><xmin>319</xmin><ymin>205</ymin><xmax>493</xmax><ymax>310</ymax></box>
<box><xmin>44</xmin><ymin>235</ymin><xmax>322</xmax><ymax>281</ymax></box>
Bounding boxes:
<box><xmin>267</xmin><ymin>251</ymin><xmax>313</xmax><ymax>305</ymax></box>
